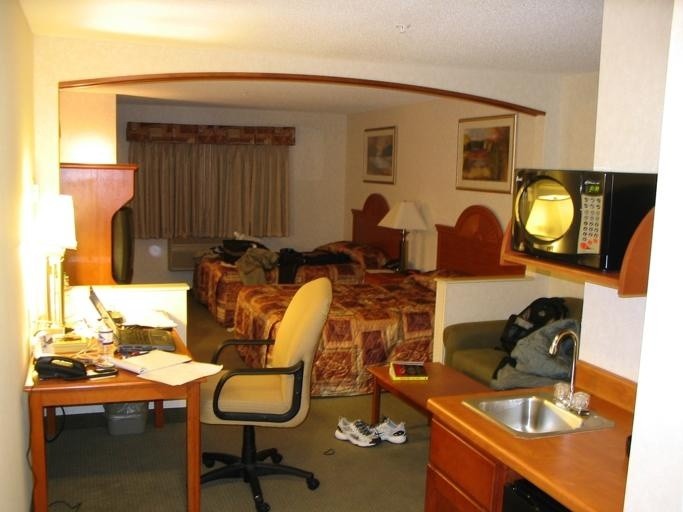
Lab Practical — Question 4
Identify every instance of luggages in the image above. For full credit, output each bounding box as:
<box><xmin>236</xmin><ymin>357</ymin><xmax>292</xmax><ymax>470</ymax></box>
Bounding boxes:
<box><xmin>222</xmin><ymin>239</ymin><xmax>269</xmax><ymax>263</ymax></box>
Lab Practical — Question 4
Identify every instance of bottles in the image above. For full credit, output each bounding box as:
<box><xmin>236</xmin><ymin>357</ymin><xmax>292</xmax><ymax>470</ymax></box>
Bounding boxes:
<box><xmin>97</xmin><ymin>313</ymin><xmax>116</xmax><ymax>369</ymax></box>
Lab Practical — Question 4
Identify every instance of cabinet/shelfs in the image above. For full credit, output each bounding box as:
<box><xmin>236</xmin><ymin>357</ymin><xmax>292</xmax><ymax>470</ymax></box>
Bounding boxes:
<box><xmin>424</xmin><ymin>417</ymin><xmax>506</xmax><ymax>512</ymax></box>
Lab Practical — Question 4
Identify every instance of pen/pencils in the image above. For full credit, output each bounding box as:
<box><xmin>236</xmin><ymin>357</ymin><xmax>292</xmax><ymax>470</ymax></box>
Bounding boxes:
<box><xmin>130</xmin><ymin>350</ymin><xmax>148</xmax><ymax>356</ymax></box>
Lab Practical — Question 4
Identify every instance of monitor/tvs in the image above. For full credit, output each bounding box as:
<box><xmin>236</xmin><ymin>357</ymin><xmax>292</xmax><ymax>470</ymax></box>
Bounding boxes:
<box><xmin>112</xmin><ymin>207</ymin><xmax>133</xmax><ymax>283</ymax></box>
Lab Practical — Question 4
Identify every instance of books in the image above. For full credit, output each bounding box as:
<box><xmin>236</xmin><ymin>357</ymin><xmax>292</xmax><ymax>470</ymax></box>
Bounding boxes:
<box><xmin>101</xmin><ymin>349</ymin><xmax>192</xmax><ymax>375</ymax></box>
<box><xmin>384</xmin><ymin>361</ymin><xmax>429</xmax><ymax>380</ymax></box>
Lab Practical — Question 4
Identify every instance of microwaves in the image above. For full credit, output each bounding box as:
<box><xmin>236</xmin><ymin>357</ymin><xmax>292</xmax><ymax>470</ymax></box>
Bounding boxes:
<box><xmin>511</xmin><ymin>167</ymin><xmax>656</xmax><ymax>275</ymax></box>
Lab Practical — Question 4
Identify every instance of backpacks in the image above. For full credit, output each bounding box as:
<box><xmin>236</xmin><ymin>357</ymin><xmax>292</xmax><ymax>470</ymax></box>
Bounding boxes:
<box><xmin>501</xmin><ymin>297</ymin><xmax>569</xmax><ymax>356</ymax></box>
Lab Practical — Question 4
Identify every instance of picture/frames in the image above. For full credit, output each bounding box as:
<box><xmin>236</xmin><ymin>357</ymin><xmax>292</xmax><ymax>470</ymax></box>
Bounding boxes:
<box><xmin>361</xmin><ymin>126</ymin><xmax>397</xmax><ymax>185</ymax></box>
<box><xmin>455</xmin><ymin>113</ymin><xmax>518</xmax><ymax>195</ymax></box>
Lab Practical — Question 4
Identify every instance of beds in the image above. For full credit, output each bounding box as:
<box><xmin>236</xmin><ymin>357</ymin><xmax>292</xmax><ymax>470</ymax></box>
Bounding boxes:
<box><xmin>192</xmin><ymin>193</ymin><xmax>402</xmax><ymax>326</ymax></box>
<box><xmin>234</xmin><ymin>205</ymin><xmax>525</xmax><ymax>399</ymax></box>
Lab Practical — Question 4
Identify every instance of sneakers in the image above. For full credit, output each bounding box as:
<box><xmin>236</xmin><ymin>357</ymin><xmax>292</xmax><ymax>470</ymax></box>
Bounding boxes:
<box><xmin>333</xmin><ymin>415</ymin><xmax>380</xmax><ymax>449</ymax></box>
<box><xmin>365</xmin><ymin>416</ymin><xmax>408</xmax><ymax>445</ymax></box>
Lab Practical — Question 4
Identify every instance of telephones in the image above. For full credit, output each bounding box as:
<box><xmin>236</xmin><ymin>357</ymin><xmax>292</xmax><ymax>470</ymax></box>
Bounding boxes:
<box><xmin>34</xmin><ymin>356</ymin><xmax>87</xmax><ymax>379</ymax></box>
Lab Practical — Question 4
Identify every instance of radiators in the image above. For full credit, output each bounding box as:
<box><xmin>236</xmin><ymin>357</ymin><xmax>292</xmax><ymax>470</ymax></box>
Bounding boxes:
<box><xmin>168</xmin><ymin>238</ymin><xmax>236</xmax><ymax>271</ymax></box>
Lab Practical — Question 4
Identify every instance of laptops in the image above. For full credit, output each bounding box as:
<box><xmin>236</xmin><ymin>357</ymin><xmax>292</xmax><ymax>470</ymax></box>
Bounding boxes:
<box><xmin>88</xmin><ymin>286</ymin><xmax>177</xmax><ymax>353</ymax></box>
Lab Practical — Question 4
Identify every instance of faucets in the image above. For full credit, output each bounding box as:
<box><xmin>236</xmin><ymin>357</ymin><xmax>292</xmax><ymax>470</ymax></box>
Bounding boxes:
<box><xmin>548</xmin><ymin>328</ymin><xmax>579</xmax><ymax>411</ymax></box>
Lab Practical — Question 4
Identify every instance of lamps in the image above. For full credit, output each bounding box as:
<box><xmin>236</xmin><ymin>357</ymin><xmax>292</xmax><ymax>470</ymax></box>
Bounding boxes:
<box><xmin>376</xmin><ymin>201</ymin><xmax>427</xmax><ymax>268</ymax></box>
<box><xmin>38</xmin><ymin>191</ymin><xmax>78</xmax><ymax>325</ymax></box>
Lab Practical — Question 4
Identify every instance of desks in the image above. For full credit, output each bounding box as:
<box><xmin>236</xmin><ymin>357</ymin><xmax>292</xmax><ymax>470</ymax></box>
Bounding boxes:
<box><xmin>24</xmin><ymin>327</ymin><xmax>208</xmax><ymax>511</ymax></box>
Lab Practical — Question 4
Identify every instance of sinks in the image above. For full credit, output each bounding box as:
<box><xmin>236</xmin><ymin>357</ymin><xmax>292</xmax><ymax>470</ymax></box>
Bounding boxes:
<box><xmin>461</xmin><ymin>391</ymin><xmax>614</xmax><ymax>440</ymax></box>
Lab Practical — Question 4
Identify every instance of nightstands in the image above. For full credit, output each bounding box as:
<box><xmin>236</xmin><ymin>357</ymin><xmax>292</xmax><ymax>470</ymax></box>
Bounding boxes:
<box><xmin>363</xmin><ymin>266</ymin><xmax>419</xmax><ymax>286</ymax></box>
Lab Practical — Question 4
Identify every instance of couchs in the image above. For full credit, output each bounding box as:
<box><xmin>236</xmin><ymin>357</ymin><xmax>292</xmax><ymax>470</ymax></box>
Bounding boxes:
<box><xmin>444</xmin><ymin>297</ymin><xmax>583</xmax><ymax>392</ymax></box>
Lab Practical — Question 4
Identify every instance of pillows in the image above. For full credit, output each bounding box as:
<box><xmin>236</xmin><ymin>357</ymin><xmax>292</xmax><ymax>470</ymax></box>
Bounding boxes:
<box><xmin>404</xmin><ymin>270</ymin><xmax>464</xmax><ymax>293</ymax></box>
<box><xmin>314</xmin><ymin>240</ymin><xmax>388</xmax><ymax>267</ymax></box>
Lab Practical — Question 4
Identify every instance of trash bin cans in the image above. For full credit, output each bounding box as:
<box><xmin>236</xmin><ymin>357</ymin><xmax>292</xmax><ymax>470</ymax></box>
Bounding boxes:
<box><xmin>103</xmin><ymin>402</ymin><xmax>148</xmax><ymax>436</ymax></box>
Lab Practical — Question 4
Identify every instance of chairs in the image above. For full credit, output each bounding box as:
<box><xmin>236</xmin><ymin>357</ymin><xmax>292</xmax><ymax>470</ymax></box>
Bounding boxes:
<box><xmin>197</xmin><ymin>277</ymin><xmax>333</xmax><ymax>511</ymax></box>
